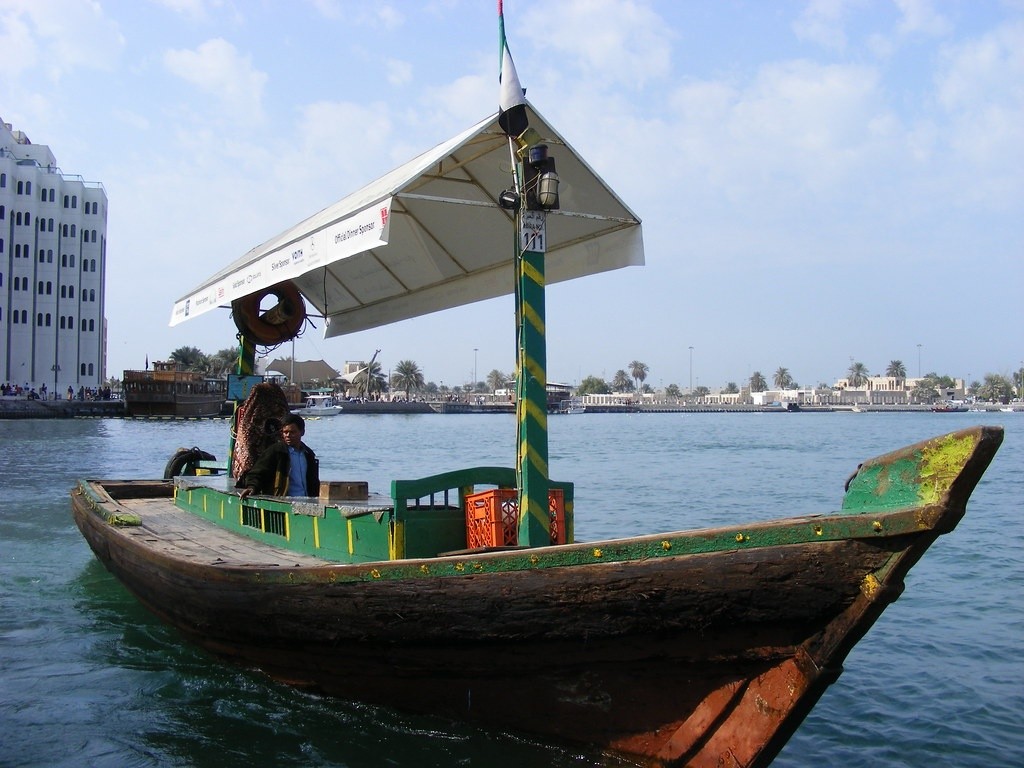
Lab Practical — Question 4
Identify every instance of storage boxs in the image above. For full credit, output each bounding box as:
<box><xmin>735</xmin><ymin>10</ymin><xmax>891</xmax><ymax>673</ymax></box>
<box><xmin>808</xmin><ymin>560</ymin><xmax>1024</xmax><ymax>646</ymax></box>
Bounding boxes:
<box><xmin>320</xmin><ymin>481</ymin><xmax>368</xmax><ymax>500</ymax></box>
<box><xmin>465</xmin><ymin>488</ymin><xmax>565</xmax><ymax>549</ymax></box>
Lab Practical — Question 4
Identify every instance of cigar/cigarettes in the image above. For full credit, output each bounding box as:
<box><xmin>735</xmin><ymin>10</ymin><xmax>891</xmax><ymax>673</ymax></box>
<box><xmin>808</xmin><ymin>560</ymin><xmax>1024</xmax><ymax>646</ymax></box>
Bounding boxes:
<box><xmin>237</xmin><ymin>492</ymin><xmax>241</xmax><ymax>496</ymax></box>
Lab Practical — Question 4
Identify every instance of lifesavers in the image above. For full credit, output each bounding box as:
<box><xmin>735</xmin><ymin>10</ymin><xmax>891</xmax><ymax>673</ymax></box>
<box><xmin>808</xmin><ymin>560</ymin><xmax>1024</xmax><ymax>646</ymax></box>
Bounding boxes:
<box><xmin>163</xmin><ymin>446</ymin><xmax>221</xmax><ymax>485</ymax></box>
<box><xmin>232</xmin><ymin>276</ymin><xmax>310</xmax><ymax>350</ymax></box>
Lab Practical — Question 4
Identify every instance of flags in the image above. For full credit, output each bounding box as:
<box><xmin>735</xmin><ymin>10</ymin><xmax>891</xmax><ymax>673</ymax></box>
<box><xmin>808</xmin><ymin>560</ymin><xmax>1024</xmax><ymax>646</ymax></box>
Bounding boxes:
<box><xmin>498</xmin><ymin>1</ymin><xmax>528</xmax><ymax>137</ymax></box>
<box><xmin>146</xmin><ymin>355</ymin><xmax>148</xmax><ymax>369</ymax></box>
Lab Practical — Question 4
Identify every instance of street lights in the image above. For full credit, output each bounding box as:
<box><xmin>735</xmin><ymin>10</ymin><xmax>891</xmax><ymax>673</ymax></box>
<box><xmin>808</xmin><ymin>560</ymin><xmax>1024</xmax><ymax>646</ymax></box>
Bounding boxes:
<box><xmin>688</xmin><ymin>346</ymin><xmax>694</xmax><ymax>393</ymax></box>
<box><xmin>473</xmin><ymin>348</ymin><xmax>478</xmax><ymax>385</ymax></box>
<box><xmin>915</xmin><ymin>343</ymin><xmax>922</xmax><ymax>378</ymax></box>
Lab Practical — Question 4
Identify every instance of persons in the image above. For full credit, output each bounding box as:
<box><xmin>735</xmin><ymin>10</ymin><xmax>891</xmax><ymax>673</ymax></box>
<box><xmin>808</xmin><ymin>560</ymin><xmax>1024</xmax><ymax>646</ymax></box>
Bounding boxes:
<box><xmin>0</xmin><ymin>382</ymin><xmax>112</xmax><ymax>402</ymax></box>
<box><xmin>240</xmin><ymin>414</ymin><xmax>320</xmax><ymax>503</ymax></box>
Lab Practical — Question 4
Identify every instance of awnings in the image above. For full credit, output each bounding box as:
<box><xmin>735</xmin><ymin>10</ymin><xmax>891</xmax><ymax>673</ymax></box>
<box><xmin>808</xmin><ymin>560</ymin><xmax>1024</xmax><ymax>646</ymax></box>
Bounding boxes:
<box><xmin>169</xmin><ymin>98</ymin><xmax>644</xmax><ymax>338</ymax></box>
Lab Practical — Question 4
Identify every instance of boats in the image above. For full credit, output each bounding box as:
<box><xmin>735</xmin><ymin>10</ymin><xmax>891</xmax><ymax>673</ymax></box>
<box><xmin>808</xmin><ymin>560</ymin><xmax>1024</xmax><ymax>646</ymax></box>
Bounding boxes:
<box><xmin>931</xmin><ymin>400</ymin><xmax>970</xmax><ymax>413</ymax></box>
<box><xmin>68</xmin><ymin>0</ymin><xmax>1004</xmax><ymax>767</ymax></box>
<box><xmin>120</xmin><ymin>359</ymin><xmax>228</xmax><ymax>418</ymax></box>
<box><xmin>290</xmin><ymin>394</ymin><xmax>343</xmax><ymax>416</ymax></box>
